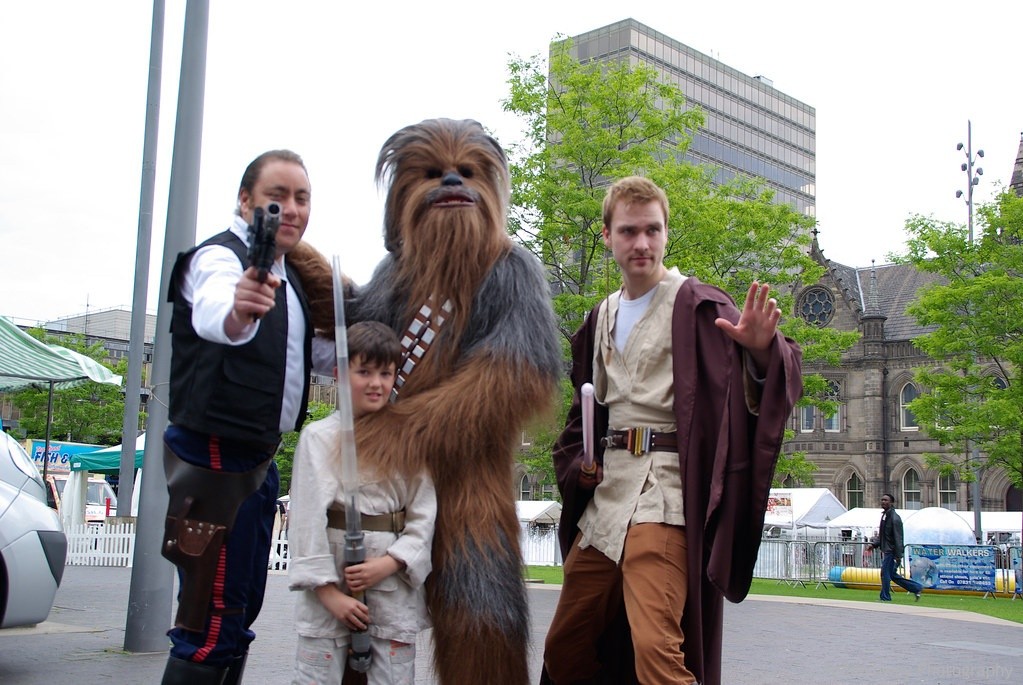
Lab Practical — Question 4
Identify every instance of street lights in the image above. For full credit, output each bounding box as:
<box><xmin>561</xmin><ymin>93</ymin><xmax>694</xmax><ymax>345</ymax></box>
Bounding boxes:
<box><xmin>955</xmin><ymin>116</ymin><xmax>984</xmax><ymax>548</ymax></box>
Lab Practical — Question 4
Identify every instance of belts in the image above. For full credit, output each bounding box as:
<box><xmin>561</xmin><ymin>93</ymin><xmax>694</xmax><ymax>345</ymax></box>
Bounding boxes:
<box><xmin>326</xmin><ymin>509</ymin><xmax>406</xmax><ymax>531</ymax></box>
<box><xmin>602</xmin><ymin>428</ymin><xmax>678</xmax><ymax>456</ymax></box>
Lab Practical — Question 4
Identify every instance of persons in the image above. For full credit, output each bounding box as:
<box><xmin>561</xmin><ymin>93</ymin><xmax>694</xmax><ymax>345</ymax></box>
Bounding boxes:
<box><xmin>269</xmin><ymin>488</ymin><xmax>291</xmax><ymax>569</ymax></box>
<box><xmin>868</xmin><ymin>493</ymin><xmax>922</xmax><ymax>602</ymax></box>
<box><xmin>292</xmin><ymin>319</ymin><xmax>437</xmax><ymax>684</ymax></box>
<box><xmin>160</xmin><ymin>150</ymin><xmax>338</xmax><ymax>684</ymax></box>
<box><xmin>870</xmin><ymin>531</ymin><xmax>879</xmax><ymax>543</ymax></box>
<box><xmin>540</xmin><ymin>177</ymin><xmax>804</xmax><ymax>684</ymax></box>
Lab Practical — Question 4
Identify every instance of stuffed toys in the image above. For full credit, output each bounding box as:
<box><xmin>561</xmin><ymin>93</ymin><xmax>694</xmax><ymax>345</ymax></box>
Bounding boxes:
<box><xmin>286</xmin><ymin>118</ymin><xmax>560</xmax><ymax>685</ymax></box>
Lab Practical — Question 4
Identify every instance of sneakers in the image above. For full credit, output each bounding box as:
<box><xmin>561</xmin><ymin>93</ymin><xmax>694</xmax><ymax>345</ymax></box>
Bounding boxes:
<box><xmin>878</xmin><ymin>598</ymin><xmax>891</xmax><ymax>602</ymax></box>
<box><xmin>915</xmin><ymin>586</ymin><xmax>923</xmax><ymax>602</ymax></box>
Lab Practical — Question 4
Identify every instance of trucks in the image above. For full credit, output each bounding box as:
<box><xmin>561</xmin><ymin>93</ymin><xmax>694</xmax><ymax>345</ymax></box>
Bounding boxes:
<box><xmin>16</xmin><ymin>439</ymin><xmax>119</xmax><ymax>534</ymax></box>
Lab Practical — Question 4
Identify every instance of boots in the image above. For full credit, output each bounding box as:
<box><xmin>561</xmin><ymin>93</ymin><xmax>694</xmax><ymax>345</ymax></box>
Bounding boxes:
<box><xmin>160</xmin><ymin>649</ymin><xmax>250</xmax><ymax>685</ymax></box>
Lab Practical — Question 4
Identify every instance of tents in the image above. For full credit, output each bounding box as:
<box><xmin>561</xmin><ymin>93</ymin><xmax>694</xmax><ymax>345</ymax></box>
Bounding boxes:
<box><xmin>517</xmin><ymin>500</ymin><xmax>562</xmax><ymax>565</ymax></box>
<box><xmin>63</xmin><ymin>432</ymin><xmax>145</xmax><ymax>523</ymax></box>
<box><xmin>764</xmin><ymin>487</ymin><xmax>847</xmax><ymax>577</ymax></box>
<box><xmin>825</xmin><ymin>508</ymin><xmax>1023</xmax><ymax>566</ymax></box>
<box><xmin>0</xmin><ymin>317</ymin><xmax>121</xmax><ymax>482</ymax></box>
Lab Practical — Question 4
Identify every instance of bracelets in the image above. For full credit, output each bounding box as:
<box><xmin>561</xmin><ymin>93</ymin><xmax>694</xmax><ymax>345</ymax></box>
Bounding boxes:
<box><xmin>231</xmin><ymin>310</ymin><xmax>239</xmax><ymax>322</ymax></box>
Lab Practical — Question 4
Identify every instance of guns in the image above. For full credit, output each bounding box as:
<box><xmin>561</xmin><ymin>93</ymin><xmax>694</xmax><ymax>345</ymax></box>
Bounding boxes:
<box><xmin>246</xmin><ymin>197</ymin><xmax>283</xmax><ymax>323</ymax></box>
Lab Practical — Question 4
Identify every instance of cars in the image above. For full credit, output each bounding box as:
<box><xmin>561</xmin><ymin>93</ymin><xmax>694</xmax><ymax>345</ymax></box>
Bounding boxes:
<box><xmin>0</xmin><ymin>429</ymin><xmax>68</xmax><ymax>631</ymax></box>
